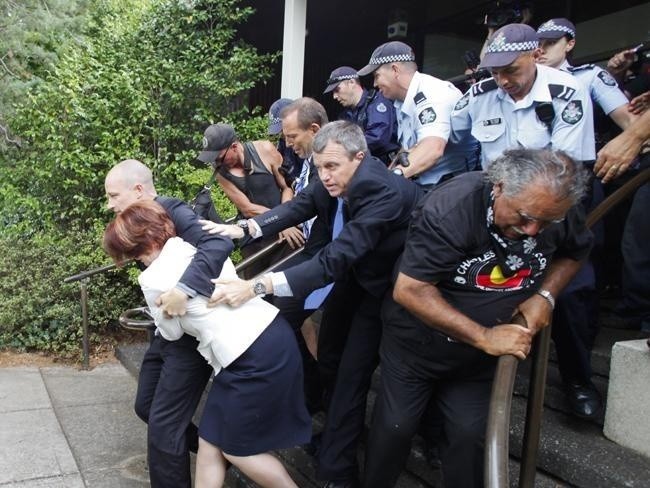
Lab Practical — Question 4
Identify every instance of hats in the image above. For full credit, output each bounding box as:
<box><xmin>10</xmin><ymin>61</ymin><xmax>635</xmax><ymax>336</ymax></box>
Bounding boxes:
<box><xmin>477</xmin><ymin>23</ymin><xmax>540</xmax><ymax>69</ymax></box>
<box><xmin>536</xmin><ymin>18</ymin><xmax>576</xmax><ymax>40</ymax></box>
<box><xmin>197</xmin><ymin>124</ymin><xmax>237</xmax><ymax>163</ymax></box>
<box><xmin>266</xmin><ymin>99</ymin><xmax>296</xmax><ymax>136</ymax></box>
<box><xmin>323</xmin><ymin>66</ymin><xmax>359</xmax><ymax>96</ymax></box>
<box><xmin>357</xmin><ymin>41</ymin><xmax>416</xmax><ymax>77</ymax></box>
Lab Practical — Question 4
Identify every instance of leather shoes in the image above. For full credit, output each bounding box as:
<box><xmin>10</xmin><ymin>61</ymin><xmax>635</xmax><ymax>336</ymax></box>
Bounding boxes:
<box><xmin>561</xmin><ymin>374</ymin><xmax>602</xmax><ymax>419</ymax></box>
<box><xmin>426</xmin><ymin>440</ymin><xmax>443</xmax><ymax>469</ymax></box>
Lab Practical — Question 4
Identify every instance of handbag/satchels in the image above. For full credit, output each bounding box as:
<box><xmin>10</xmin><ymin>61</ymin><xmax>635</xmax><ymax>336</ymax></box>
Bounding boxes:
<box><xmin>190</xmin><ymin>189</ymin><xmax>227</xmax><ymax>224</ymax></box>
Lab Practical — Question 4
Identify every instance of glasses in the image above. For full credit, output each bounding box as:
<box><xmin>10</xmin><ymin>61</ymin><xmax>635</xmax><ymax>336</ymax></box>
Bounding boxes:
<box><xmin>214</xmin><ymin>148</ymin><xmax>229</xmax><ymax>164</ymax></box>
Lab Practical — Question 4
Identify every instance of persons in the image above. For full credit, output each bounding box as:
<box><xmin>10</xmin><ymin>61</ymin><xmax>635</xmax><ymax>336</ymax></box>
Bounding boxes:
<box><xmin>177</xmin><ymin>1</ymin><xmax>650</xmax><ymax>488</ymax></box>
<box><xmin>98</xmin><ymin>198</ymin><xmax>313</xmax><ymax>488</ymax></box>
<box><xmin>104</xmin><ymin>157</ymin><xmax>240</xmax><ymax>487</ymax></box>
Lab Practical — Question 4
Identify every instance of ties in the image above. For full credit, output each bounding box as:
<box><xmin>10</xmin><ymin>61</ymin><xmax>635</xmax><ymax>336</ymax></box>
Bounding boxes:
<box><xmin>294</xmin><ymin>158</ymin><xmax>310</xmax><ymax>241</ymax></box>
<box><xmin>303</xmin><ymin>195</ymin><xmax>346</xmax><ymax>311</ymax></box>
